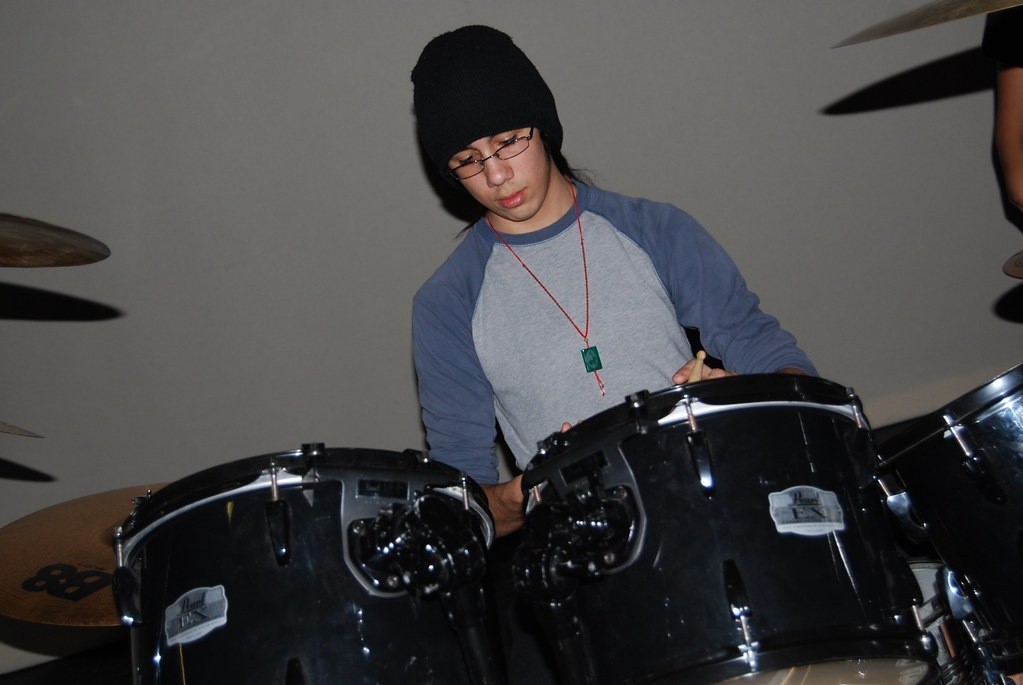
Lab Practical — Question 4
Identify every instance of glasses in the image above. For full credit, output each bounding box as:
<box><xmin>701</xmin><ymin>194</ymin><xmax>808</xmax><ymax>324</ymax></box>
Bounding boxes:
<box><xmin>446</xmin><ymin>126</ymin><xmax>534</xmax><ymax>181</ymax></box>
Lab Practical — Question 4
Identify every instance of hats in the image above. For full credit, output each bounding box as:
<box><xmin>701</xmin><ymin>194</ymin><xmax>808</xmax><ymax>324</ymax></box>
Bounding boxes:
<box><xmin>411</xmin><ymin>24</ymin><xmax>563</xmax><ymax>174</ymax></box>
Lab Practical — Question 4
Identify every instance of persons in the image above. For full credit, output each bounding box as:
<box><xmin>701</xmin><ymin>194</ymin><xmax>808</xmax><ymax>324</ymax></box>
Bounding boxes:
<box><xmin>985</xmin><ymin>4</ymin><xmax>1023</xmax><ymax>245</ymax></box>
<box><xmin>410</xmin><ymin>25</ymin><xmax>821</xmax><ymax>539</ymax></box>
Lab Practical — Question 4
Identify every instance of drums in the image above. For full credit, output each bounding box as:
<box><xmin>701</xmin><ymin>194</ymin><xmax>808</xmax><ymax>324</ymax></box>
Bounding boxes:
<box><xmin>880</xmin><ymin>359</ymin><xmax>1023</xmax><ymax>679</ymax></box>
<box><xmin>107</xmin><ymin>442</ymin><xmax>500</xmax><ymax>685</ymax></box>
<box><xmin>515</xmin><ymin>371</ymin><xmax>931</xmax><ymax>685</ymax></box>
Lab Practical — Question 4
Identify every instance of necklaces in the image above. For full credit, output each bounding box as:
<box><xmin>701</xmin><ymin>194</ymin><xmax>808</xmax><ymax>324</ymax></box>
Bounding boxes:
<box><xmin>487</xmin><ymin>176</ymin><xmax>606</xmax><ymax>396</ymax></box>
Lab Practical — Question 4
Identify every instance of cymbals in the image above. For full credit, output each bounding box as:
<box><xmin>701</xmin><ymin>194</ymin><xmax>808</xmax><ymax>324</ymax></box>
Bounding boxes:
<box><xmin>0</xmin><ymin>421</ymin><xmax>46</xmax><ymax>438</ymax></box>
<box><xmin>0</xmin><ymin>211</ymin><xmax>113</xmax><ymax>268</ymax></box>
<box><xmin>0</xmin><ymin>479</ymin><xmax>176</xmax><ymax>627</ymax></box>
<box><xmin>829</xmin><ymin>0</ymin><xmax>1023</xmax><ymax>51</ymax></box>
